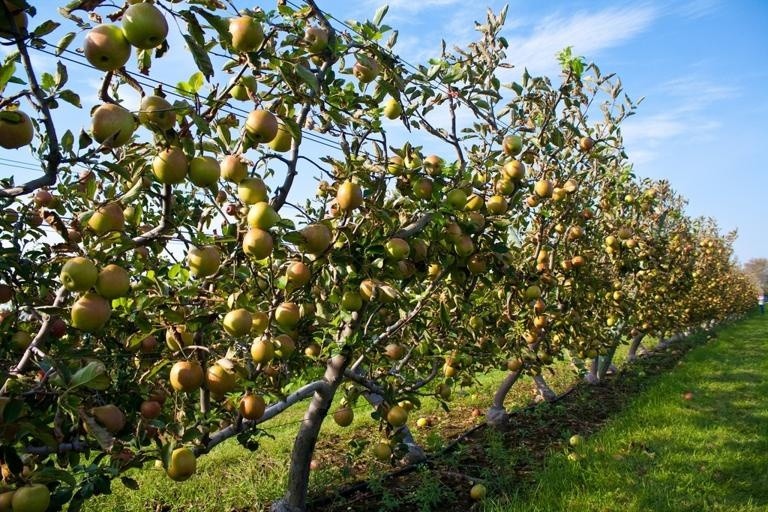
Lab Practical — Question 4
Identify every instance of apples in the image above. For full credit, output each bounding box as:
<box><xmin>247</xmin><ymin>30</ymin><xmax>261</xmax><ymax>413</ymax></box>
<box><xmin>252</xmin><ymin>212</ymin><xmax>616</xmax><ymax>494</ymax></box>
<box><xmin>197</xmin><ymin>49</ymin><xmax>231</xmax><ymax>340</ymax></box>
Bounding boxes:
<box><xmin>0</xmin><ymin>0</ymin><xmax>752</xmax><ymax>512</ymax></box>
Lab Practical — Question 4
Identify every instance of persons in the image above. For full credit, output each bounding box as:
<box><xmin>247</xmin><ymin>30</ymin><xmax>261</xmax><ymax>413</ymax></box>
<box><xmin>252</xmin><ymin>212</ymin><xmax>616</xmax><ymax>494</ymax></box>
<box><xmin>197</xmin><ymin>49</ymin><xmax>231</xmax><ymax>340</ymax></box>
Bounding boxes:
<box><xmin>757</xmin><ymin>292</ymin><xmax>765</xmax><ymax>317</ymax></box>
